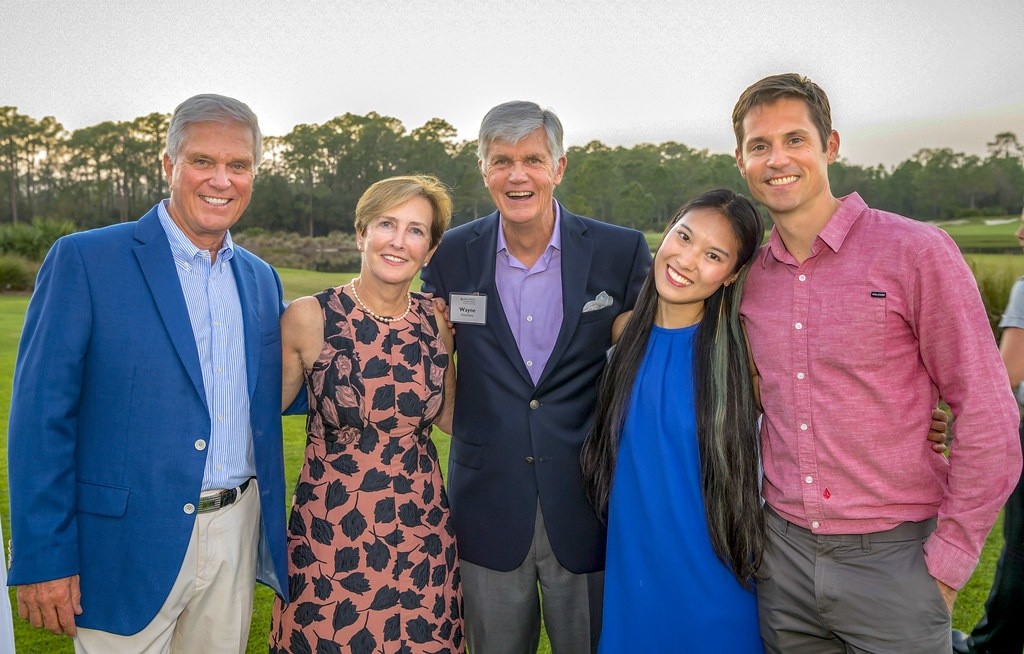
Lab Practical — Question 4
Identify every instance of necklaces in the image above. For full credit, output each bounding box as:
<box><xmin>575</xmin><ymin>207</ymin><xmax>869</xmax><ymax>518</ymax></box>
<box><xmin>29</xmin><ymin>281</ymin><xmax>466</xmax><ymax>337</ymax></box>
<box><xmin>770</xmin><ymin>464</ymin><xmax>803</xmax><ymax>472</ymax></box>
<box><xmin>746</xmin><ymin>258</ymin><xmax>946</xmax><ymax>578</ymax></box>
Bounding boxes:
<box><xmin>347</xmin><ymin>279</ymin><xmax>415</xmax><ymax>323</ymax></box>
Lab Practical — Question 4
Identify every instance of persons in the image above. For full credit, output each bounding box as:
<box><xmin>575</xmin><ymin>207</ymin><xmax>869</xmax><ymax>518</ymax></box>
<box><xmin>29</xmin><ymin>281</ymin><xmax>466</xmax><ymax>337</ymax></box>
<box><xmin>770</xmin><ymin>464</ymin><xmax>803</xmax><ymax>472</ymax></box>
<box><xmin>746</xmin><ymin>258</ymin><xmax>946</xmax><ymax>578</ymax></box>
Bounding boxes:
<box><xmin>277</xmin><ymin>172</ymin><xmax>475</xmax><ymax>652</ymax></box>
<box><xmin>3</xmin><ymin>92</ymin><xmax>307</xmax><ymax>654</ymax></box>
<box><xmin>579</xmin><ymin>184</ymin><xmax>952</xmax><ymax>654</ymax></box>
<box><xmin>943</xmin><ymin>229</ymin><xmax>1024</xmax><ymax>654</ymax></box>
<box><xmin>728</xmin><ymin>71</ymin><xmax>1024</xmax><ymax>651</ymax></box>
<box><xmin>419</xmin><ymin>97</ymin><xmax>654</xmax><ymax>652</ymax></box>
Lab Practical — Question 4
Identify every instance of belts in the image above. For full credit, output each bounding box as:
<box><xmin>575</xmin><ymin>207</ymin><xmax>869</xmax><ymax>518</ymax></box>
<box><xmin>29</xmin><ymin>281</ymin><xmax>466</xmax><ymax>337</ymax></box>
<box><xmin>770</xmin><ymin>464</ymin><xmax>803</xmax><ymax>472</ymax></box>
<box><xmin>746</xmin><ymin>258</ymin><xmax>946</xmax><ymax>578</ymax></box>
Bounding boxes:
<box><xmin>197</xmin><ymin>476</ymin><xmax>257</xmax><ymax>515</ymax></box>
